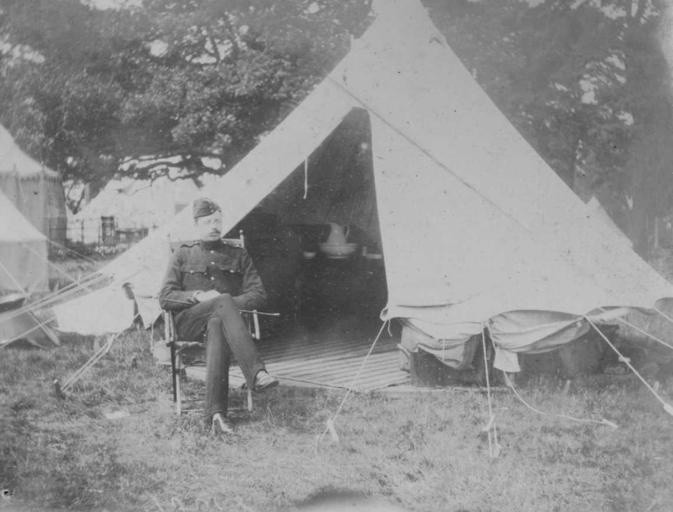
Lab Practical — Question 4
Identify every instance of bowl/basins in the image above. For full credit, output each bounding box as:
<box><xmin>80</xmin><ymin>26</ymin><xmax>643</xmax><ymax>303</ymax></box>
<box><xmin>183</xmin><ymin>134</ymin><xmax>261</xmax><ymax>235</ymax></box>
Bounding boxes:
<box><xmin>320</xmin><ymin>243</ymin><xmax>358</xmax><ymax>260</ymax></box>
<box><xmin>302</xmin><ymin>251</ymin><xmax>317</xmax><ymax>260</ymax></box>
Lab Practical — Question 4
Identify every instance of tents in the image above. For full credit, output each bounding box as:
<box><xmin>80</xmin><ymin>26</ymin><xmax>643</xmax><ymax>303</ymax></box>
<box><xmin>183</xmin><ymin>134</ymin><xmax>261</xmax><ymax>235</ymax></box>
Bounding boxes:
<box><xmin>48</xmin><ymin>0</ymin><xmax>671</xmax><ymax>402</ymax></box>
<box><xmin>0</xmin><ymin>191</ymin><xmax>56</xmax><ymax>310</ymax></box>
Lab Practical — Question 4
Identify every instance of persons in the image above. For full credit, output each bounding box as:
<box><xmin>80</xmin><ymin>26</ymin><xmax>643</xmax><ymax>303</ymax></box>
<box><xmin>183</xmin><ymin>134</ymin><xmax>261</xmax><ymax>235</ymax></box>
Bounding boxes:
<box><xmin>157</xmin><ymin>196</ymin><xmax>281</xmax><ymax>439</ymax></box>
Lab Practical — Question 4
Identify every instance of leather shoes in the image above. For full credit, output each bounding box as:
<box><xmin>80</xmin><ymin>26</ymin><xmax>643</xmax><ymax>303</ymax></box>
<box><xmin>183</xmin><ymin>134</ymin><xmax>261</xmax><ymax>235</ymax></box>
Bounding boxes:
<box><xmin>208</xmin><ymin>411</ymin><xmax>233</xmax><ymax>435</ymax></box>
<box><xmin>252</xmin><ymin>370</ymin><xmax>278</xmax><ymax>393</ymax></box>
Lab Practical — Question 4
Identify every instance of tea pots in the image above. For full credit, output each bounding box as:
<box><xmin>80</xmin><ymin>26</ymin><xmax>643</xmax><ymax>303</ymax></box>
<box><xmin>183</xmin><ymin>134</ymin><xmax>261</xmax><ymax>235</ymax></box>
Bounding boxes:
<box><xmin>326</xmin><ymin>221</ymin><xmax>350</xmax><ymax>252</ymax></box>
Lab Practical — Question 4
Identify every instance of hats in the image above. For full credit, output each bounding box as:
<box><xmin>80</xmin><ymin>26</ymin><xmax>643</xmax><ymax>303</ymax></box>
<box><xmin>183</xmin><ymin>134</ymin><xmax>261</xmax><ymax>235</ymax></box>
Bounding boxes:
<box><xmin>191</xmin><ymin>196</ymin><xmax>220</xmax><ymax>219</ymax></box>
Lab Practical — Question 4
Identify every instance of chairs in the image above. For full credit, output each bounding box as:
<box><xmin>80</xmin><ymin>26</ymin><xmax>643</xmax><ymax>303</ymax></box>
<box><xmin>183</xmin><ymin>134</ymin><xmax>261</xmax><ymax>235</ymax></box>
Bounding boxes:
<box><xmin>163</xmin><ymin>229</ymin><xmax>261</xmax><ymax>415</ymax></box>
